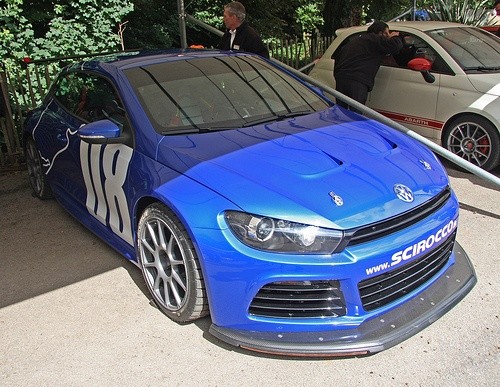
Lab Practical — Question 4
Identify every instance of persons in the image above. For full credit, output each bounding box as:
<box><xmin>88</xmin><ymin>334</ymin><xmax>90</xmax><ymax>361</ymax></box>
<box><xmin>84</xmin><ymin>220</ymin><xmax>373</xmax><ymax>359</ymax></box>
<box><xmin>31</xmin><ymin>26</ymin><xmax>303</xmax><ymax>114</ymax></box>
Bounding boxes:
<box><xmin>213</xmin><ymin>1</ymin><xmax>269</xmax><ymax>59</ymax></box>
<box><xmin>333</xmin><ymin>21</ymin><xmax>403</xmax><ymax>115</ymax></box>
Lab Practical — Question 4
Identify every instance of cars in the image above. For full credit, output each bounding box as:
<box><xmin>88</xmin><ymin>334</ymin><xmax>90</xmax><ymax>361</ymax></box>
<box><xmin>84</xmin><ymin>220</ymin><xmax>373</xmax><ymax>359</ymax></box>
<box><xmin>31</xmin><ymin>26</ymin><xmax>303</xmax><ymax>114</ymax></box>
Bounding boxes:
<box><xmin>305</xmin><ymin>20</ymin><xmax>500</xmax><ymax>174</ymax></box>
<box><xmin>21</xmin><ymin>48</ymin><xmax>479</xmax><ymax>358</ymax></box>
